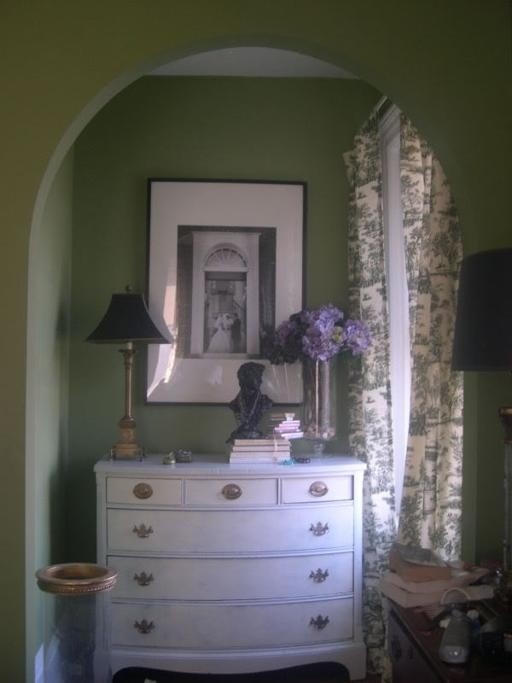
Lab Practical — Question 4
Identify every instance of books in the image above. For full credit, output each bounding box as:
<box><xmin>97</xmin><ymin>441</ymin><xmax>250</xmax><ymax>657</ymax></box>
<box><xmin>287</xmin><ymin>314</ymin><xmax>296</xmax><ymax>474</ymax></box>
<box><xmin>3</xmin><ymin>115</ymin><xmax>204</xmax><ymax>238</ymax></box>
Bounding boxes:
<box><xmin>227</xmin><ymin>411</ymin><xmax>304</xmax><ymax>467</ymax></box>
<box><xmin>380</xmin><ymin>544</ymin><xmax>496</xmax><ymax>609</ymax></box>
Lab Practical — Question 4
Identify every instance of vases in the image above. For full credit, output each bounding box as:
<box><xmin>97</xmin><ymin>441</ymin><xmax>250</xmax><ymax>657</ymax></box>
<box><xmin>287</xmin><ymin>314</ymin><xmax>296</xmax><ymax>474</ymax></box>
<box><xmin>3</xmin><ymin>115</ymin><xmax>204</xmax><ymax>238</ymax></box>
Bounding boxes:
<box><xmin>302</xmin><ymin>361</ymin><xmax>336</xmax><ymax>441</ymax></box>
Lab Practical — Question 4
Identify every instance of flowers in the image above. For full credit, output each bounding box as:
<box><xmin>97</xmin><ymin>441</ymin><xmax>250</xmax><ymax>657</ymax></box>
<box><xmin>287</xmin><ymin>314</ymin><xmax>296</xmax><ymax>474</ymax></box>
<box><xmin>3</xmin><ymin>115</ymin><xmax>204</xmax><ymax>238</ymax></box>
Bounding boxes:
<box><xmin>264</xmin><ymin>303</ymin><xmax>371</xmax><ymax>433</ymax></box>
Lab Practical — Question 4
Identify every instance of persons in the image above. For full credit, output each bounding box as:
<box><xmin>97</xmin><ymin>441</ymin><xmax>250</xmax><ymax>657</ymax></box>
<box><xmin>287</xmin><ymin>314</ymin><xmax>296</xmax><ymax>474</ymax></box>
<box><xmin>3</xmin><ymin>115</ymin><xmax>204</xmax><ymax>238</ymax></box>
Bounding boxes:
<box><xmin>228</xmin><ymin>362</ymin><xmax>276</xmax><ymax>430</ymax></box>
<box><xmin>205</xmin><ymin>311</ymin><xmax>243</xmax><ymax>354</ymax></box>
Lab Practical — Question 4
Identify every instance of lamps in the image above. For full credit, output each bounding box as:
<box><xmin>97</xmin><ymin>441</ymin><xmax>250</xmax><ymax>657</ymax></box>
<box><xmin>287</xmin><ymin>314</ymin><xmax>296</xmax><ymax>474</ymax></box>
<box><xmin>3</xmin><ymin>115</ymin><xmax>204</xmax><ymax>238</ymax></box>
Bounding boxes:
<box><xmin>82</xmin><ymin>290</ymin><xmax>171</xmax><ymax>463</ymax></box>
<box><xmin>447</xmin><ymin>249</ymin><xmax>510</xmax><ymax>444</ymax></box>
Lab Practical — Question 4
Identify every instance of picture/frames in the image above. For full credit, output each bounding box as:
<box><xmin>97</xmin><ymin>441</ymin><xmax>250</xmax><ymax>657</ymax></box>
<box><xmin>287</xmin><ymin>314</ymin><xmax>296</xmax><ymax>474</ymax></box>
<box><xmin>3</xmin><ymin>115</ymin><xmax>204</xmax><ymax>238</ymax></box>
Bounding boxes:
<box><xmin>143</xmin><ymin>175</ymin><xmax>309</xmax><ymax>408</ymax></box>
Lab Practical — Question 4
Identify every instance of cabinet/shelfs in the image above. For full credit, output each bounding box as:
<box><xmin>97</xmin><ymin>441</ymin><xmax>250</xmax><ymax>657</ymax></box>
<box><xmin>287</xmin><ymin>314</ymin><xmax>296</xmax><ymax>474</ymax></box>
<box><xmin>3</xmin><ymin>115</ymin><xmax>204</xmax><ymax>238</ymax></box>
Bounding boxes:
<box><xmin>88</xmin><ymin>447</ymin><xmax>367</xmax><ymax>678</ymax></box>
<box><xmin>385</xmin><ymin>594</ymin><xmax>509</xmax><ymax>681</ymax></box>
